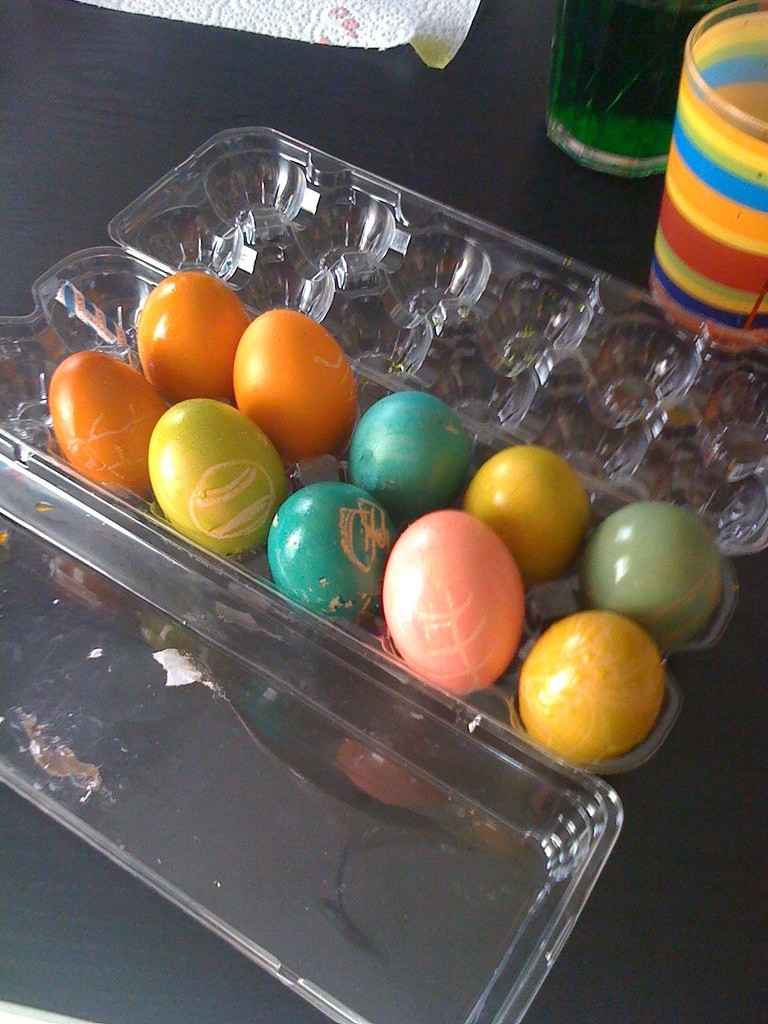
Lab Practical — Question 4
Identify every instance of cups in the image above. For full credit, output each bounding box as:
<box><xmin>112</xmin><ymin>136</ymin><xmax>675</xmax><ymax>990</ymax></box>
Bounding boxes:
<box><xmin>653</xmin><ymin>0</ymin><xmax>768</xmax><ymax>351</ymax></box>
<box><xmin>544</xmin><ymin>0</ymin><xmax>733</xmax><ymax>178</ymax></box>
